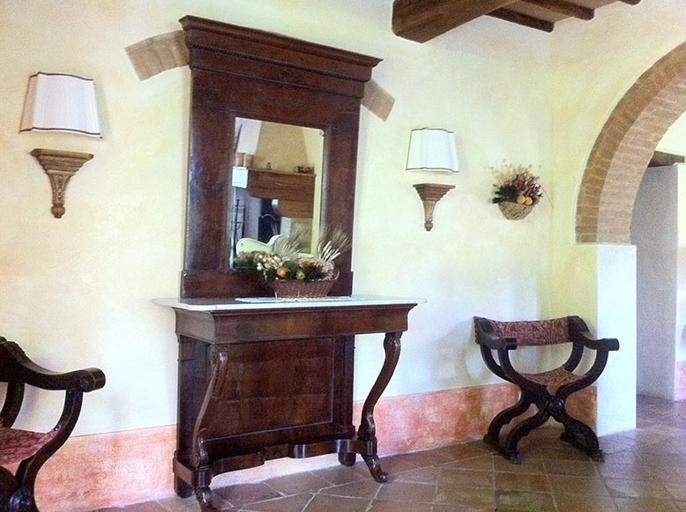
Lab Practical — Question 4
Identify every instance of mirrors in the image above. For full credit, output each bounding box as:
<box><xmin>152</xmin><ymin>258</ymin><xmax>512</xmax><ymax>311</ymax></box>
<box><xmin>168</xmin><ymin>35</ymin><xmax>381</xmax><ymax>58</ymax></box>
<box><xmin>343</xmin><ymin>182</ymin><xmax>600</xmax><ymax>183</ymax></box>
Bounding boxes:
<box><xmin>224</xmin><ymin>118</ymin><xmax>328</xmax><ymax>273</ymax></box>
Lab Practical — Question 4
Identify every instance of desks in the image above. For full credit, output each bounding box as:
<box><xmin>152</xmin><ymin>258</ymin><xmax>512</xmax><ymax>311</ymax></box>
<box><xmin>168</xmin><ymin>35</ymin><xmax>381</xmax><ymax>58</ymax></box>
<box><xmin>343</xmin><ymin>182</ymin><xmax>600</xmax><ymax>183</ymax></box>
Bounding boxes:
<box><xmin>151</xmin><ymin>295</ymin><xmax>425</xmax><ymax>512</ymax></box>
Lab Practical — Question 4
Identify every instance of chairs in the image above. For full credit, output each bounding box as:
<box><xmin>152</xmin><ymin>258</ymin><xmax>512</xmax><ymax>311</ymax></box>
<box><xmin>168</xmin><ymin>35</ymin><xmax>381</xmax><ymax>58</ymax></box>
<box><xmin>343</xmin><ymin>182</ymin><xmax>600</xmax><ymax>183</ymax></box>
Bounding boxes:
<box><xmin>469</xmin><ymin>316</ymin><xmax>619</xmax><ymax>465</ymax></box>
<box><xmin>0</xmin><ymin>334</ymin><xmax>103</xmax><ymax>512</ymax></box>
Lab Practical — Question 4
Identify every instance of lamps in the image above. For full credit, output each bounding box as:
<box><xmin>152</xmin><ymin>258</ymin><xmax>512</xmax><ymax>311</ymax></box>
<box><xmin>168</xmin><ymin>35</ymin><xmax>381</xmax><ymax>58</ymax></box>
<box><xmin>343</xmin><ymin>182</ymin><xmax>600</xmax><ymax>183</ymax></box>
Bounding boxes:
<box><xmin>405</xmin><ymin>126</ymin><xmax>460</xmax><ymax>234</ymax></box>
<box><xmin>19</xmin><ymin>70</ymin><xmax>103</xmax><ymax>218</ymax></box>
